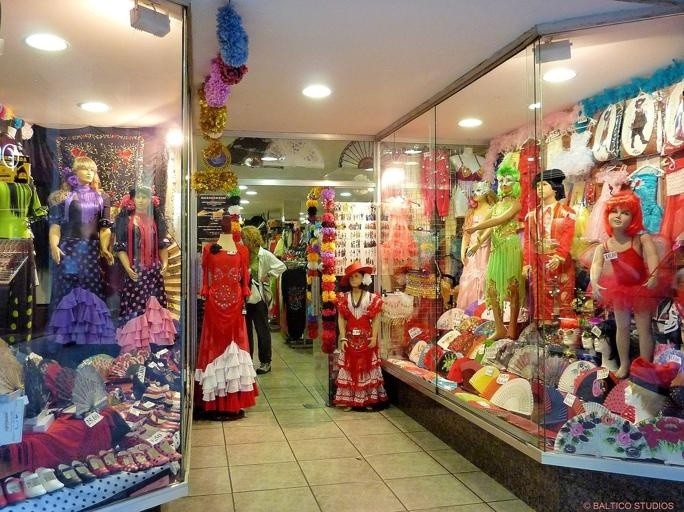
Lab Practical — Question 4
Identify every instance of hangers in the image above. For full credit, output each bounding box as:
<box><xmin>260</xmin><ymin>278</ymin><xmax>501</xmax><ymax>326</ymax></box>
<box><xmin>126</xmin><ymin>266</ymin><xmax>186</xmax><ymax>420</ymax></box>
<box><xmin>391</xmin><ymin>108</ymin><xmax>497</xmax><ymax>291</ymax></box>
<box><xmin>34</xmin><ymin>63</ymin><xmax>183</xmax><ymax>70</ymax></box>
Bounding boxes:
<box><xmin>604</xmin><ymin>162</ymin><xmax>627</xmax><ymax>172</ymax></box>
<box><xmin>660</xmin><ymin>152</ymin><xmax>674</xmax><ymax>168</ymax></box>
<box><xmin>624</xmin><ymin>156</ymin><xmax>668</xmax><ymax>183</ymax></box>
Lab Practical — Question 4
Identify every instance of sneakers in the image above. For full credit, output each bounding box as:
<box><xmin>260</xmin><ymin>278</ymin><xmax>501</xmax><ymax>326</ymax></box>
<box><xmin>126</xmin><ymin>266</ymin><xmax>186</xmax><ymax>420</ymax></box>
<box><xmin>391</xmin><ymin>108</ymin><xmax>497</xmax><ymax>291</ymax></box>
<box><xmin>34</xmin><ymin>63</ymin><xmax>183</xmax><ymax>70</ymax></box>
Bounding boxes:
<box><xmin>257</xmin><ymin>363</ymin><xmax>271</xmax><ymax>374</ymax></box>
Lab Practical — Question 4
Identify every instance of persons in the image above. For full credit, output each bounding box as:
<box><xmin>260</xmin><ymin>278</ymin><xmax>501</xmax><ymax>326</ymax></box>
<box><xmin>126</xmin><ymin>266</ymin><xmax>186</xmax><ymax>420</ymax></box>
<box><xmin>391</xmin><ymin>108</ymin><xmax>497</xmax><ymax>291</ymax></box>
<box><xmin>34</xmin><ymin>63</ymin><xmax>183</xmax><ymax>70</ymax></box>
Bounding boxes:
<box><xmin>592</xmin><ymin>321</ymin><xmax>620</xmax><ymax>372</ymax></box>
<box><xmin>195</xmin><ymin>233</ymin><xmax>259</xmax><ymax>419</ymax></box>
<box><xmin>456</xmin><ymin>179</ymin><xmax>496</xmax><ymax>313</ymax></box>
<box><xmin>578</xmin><ymin>317</ymin><xmax>594</xmax><ymax>351</ymax></box>
<box><xmin>520</xmin><ymin>169</ymin><xmax>577</xmax><ymax>333</ymax></box>
<box><xmin>0</xmin><ymin>160</ymin><xmax>48</xmax><ymax>354</ymax></box>
<box><xmin>590</xmin><ymin>191</ymin><xmax>659</xmax><ymax>378</ymax></box>
<box><xmin>465</xmin><ymin>156</ymin><xmax>522</xmax><ymax>344</ymax></box>
<box><xmin>113</xmin><ymin>182</ymin><xmax>171</xmax><ymax>328</ymax></box>
<box><xmin>242</xmin><ymin>224</ymin><xmax>287</xmax><ymax>373</ymax></box>
<box><xmin>47</xmin><ymin>156</ymin><xmax>114</xmax><ymax>348</ymax></box>
<box><xmin>560</xmin><ymin>315</ymin><xmax>580</xmax><ymax>351</ymax></box>
<box><xmin>334</xmin><ymin>262</ymin><xmax>390</xmax><ymax>413</ymax></box>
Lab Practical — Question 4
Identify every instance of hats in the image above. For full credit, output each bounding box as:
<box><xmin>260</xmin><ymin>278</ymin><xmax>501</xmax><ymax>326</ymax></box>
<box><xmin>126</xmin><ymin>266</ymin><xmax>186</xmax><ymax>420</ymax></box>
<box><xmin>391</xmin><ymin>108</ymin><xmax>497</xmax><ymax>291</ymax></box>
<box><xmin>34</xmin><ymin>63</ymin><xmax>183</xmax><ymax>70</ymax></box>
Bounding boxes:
<box><xmin>630</xmin><ymin>357</ymin><xmax>680</xmax><ymax>397</ymax></box>
<box><xmin>340</xmin><ymin>262</ymin><xmax>372</xmax><ymax>286</ymax></box>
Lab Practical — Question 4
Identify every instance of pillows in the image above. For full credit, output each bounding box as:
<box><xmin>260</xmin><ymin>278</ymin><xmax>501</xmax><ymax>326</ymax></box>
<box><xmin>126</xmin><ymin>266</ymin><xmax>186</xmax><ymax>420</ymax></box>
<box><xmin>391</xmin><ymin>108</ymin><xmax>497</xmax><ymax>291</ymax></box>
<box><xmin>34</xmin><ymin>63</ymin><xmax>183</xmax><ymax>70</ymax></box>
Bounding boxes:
<box><xmin>618</xmin><ymin>93</ymin><xmax>662</xmax><ymax>161</ymax></box>
<box><xmin>660</xmin><ymin>81</ymin><xmax>683</xmax><ymax>157</ymax></box>
<box><xmin>592</xmin><ymin>100</ymin><xmax>622</xmax><ymax>163</ymax></box>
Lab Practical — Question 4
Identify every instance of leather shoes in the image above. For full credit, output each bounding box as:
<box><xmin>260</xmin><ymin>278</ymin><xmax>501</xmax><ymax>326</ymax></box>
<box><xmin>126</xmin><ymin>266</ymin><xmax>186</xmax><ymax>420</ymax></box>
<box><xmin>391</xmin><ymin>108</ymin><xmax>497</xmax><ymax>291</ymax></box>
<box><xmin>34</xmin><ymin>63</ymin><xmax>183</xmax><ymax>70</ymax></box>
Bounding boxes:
<box><xmin>85</xmin><ymin>455</ymin><xmax>110</xmax><ymax>477</ymax></box>
<box><xmin>153</xmin><ymin>442</ymin><xmax>182</xmax><ymax>462</ymax></box>
<box><xmin>2</xmin><ymin>477</ymin><xmax>25</xmax><ymax>505</ymax></box>
<box><xmin>20</xmin><ymin>471</ymin><xmax>47</xmax><ymax>498</ymax></box>
<box><xmin>71</xmin><ymin>461</ymin><xmax>96</xmax><ymax>483</ymax></box>
<box><xmin>54</xmin><ymin>464</ymin><xmax>82</xmax><ymax>488</ymax></box>
<box><xmin>35</xmin><ymin>467</ymin><xmax>64</xmax><ymax>493</ymax></box>
<box><xmin>98</xmin><ymin>450</ymin><xmax>123</xmax><ymax>474</ymax></box>
<box><xmin>0</xmin><ymin>485</ymin><xmax>7</xmax><ymax>508</ymax></box>
<box><xmin>127</xmin><ymin>447</ymin><xmax>153</xmax><ymax>470</ymax></box>
<box><xmin>118</xmin><ymin>450</ymin><xmax>139</xmax><ymax>473</ymax></box>
<box><xmin>119</xmin><ymin>384</ymin><xmax>180</xmax><ymax>446</ymax></box>
<box><xmin>138</xmin><ymin>444</ymin><xmax>169</xmax><ymax>467</ymax></box>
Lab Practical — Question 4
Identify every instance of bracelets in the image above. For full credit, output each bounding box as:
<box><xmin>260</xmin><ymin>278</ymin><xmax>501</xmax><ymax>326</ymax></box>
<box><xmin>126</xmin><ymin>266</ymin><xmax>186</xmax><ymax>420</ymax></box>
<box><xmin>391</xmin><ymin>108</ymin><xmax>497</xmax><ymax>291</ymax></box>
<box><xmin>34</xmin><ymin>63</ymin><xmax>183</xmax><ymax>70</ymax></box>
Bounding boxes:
<box><xmin>339</xmin><ymin>338</ymin><xmax>348</xmax><ymax>342</ymax></box>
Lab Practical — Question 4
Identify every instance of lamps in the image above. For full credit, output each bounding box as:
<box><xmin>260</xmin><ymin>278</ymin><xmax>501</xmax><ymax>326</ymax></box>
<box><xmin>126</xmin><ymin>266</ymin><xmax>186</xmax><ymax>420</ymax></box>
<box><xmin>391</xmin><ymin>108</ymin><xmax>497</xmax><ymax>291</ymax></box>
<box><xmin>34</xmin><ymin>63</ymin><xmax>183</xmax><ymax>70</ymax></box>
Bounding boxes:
<box><xmin>130</xmin><ymin>0</ymin><xmax>171</xmax><ymax>39</ymax></box>
<box><xmin>534</xmin><ymin>34</ymin><xmax>572</xmax><ymax>66</ymax></box>
